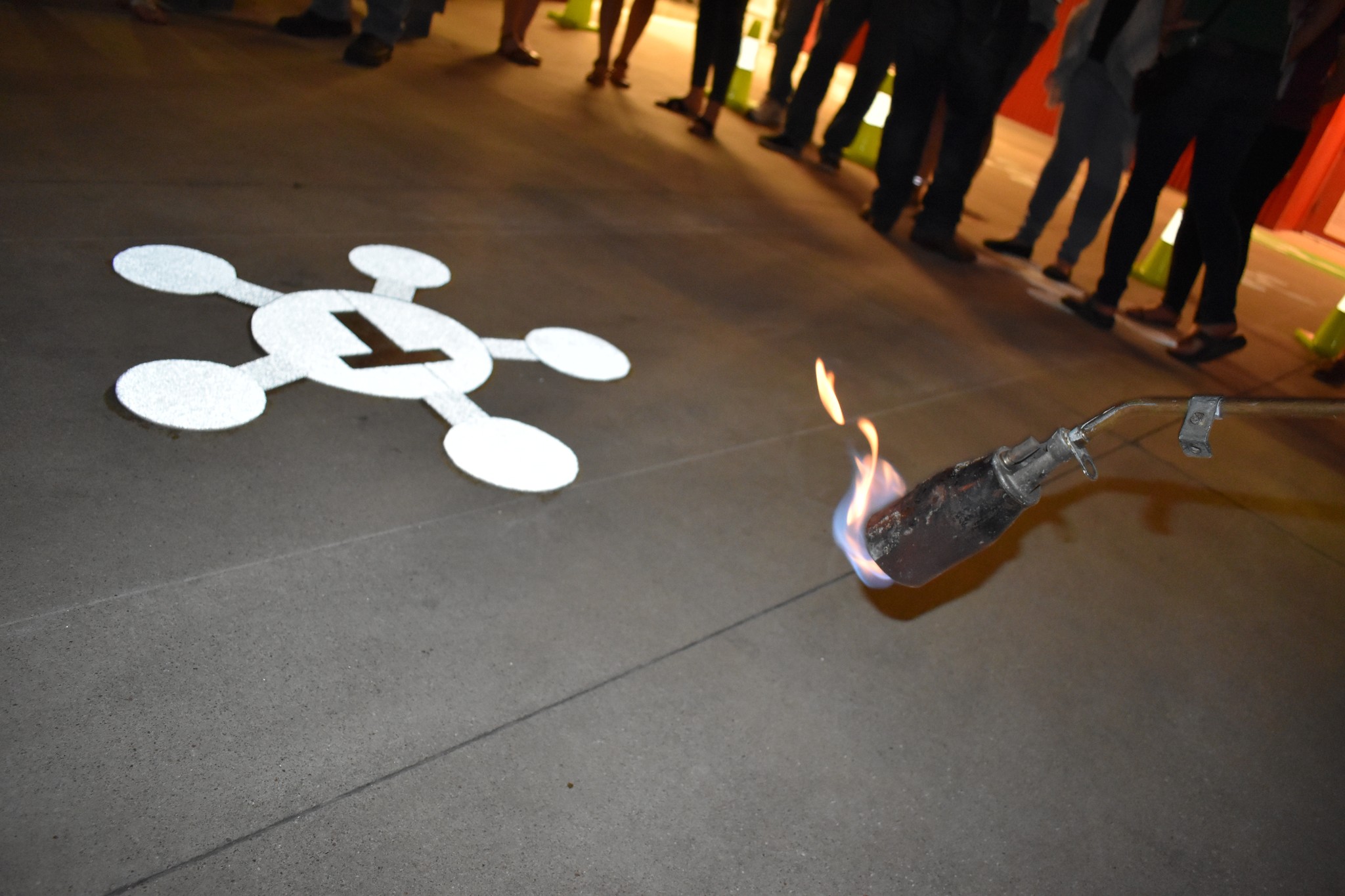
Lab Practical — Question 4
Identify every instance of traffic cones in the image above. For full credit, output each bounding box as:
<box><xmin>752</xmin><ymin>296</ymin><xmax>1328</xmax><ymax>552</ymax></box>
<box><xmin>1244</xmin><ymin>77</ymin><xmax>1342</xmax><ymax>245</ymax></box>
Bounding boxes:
<box><xmin>1132</xmin><ymin>208</ymin><xmax>1183</xmax><ymax>288</ymax></box>
<box><xmin>545</xmin><ymin>0</ymin><xmax>602</xmax><ymax>32</ymax></box>
<box><xmin>705</xmin><ymin>19</ymin><xmax>761</xmax><ymax>117</ymax></box>
<box><xmin>1295</xmin><ymin>294</ymin><xmax>1345</xmax><ymax>359</ymax></box>
<box><xmin>840</xmin><ymin>71</ymin><xmax>896</xmax><ymax>170</ymax></box>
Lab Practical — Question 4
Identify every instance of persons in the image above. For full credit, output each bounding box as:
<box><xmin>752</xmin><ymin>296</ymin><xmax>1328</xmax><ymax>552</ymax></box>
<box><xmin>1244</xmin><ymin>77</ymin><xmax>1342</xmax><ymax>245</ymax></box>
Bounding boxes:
<box><xmin>399</xmin><ymin>0</ymin><xmax>445</xmax><ymax>40</ymax></box>
<box><xmin>655</xmin><ymin>0</ymin><xmax>749</xmax><ymax>137</ymax></box>
<box><xmin>1126</xmin><ymin>0</ymin><xmax>1345</xmax><ymax>327</ymax></box>
<box><xmin>499</xmin><ymin>0</ymin><xmax>542</xmax><ymax>66</ymax></box>
<box><xmin>744</xmin><ymin>0</ymin><xmax>819</xmax><ymax>126</ymax></box>
<box><xmin>759</xmin><ymin>0</ymin><xmax>893</xmax><ymax>169</ymax></box>
<box><xmin>859</xmin><ymin>0</ymin><xmax>1055</xmax><ymax>264</ymax></box>
<box><xmin>984</xmin><ymin>0</ymin><xmax>1167</xmax><ymax>283</ymax></box>
<box><xmin>275</xmin><ymin>0</ymin><xmax>407</xmax><ymax>68</ymax></box>
<box><xmin>589</xmin><ymin>0</ymin><xmax>656</xmax><ymax>87</ymax></box>
<box><xmin>1063</xmin><ymin>0</ymin><xmax>1345</xmax><ymax>365</ymax></box>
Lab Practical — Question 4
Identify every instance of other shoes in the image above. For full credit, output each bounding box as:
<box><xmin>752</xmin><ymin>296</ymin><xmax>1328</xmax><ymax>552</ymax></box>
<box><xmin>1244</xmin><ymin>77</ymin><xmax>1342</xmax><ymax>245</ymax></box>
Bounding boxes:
<box><xmin>657</xmin><ymin>98</ymin><xmax>701</xmax><ymax>120</ymax></box>
<box><xmin>819</xmin><ymin>145</ymin><xmax>843</xmax><ymax>171</ymax></box>
<box><xmin>588</xmin><ymin>60</ymin><xmax>608</xmax><ymax>85</ymax></box>
<box><xmin>743</xmin><ymin>107</ymin><xmax>780</xmax><ymax>129</ymax></box>
<box><xmin>343</xmin><ymin>32</ymin><xmax>392</xmax><ymax>68</ymax></box>
<box><xmin>404</xmin><ymin>9</ymin><xmax>432</xmax><ymax>36</ymax></box>
<box><xmin>1312</xmin><ymin>361</ymin><xmax>1345</xmax><ymax>385</ymax></box>
<box><xmin>912</xmin><ymin>226</ymin><xmax>977</xmax><ymax>263</ymax></box>
<box><xmin>499</xmin><ymin>37</ymin><xmax>542</xmax><ymax>65</ymax></box>
<box><xmin>276</xmin><ymin>11</ymin><xmax>351</xmax><ymax>40</ymax></box>
<box><xmin>611</xmin><ymin>59</ymin><xmax>630</xmax><ymax>87</ymax></box>
<box><xmin>1044</xmin><ymin>265</ymin><xmax>1073</xmax><ymax>282</ymax></box>
<box><xmin>984</xmin><ymin>239</ymin><xmax>1033</xmax><ymax>260</ymax></box>
<box><xmin>688</xmin><ymin>118</ymin><xmax>713</xmax><ymax>139</ymax></box>
<box><xmin>128</xmin><ymin>1</ymin><xmax>166</xmax><ymax>25</ymax></box>
<box><xmin>860</xmin><ymin>207</ymin><xmax>897</xmax><ymax>234</ymax></box>
<box><xmin>1126</xmin><ymin>306</ymin><xmax>1181</xmax><ymax>328</ymax></box>
<box><xmin>760</xmin><ymin>134</ymin><xmax>803</xmax><ymax>158</ymax></box>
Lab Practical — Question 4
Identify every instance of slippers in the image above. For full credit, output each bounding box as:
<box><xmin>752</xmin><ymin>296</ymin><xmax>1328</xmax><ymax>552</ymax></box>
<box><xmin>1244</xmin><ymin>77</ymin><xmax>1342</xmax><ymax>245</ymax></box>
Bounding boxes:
<box><xmin>1062</xmin><ymin>295</ymin><xmax>1115</xmax><ymax>331</ymax></box>
<box><xmin>1168</xmin><ymin>332</ymin><xmax>1247</xmax><ymax>364</ymax></box>
